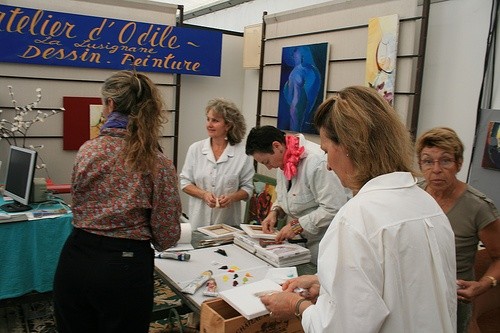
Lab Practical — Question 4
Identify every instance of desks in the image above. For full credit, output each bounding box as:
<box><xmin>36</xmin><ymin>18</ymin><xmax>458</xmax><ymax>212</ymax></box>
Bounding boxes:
<box><xmin>154</xmin><ymin>243</ymin><xmax>317</xmax><ymax>333</ymax></box>
<box><xmin>0</xmin><ymin>181</ymin><xmax>75</xmax><ymax>299</ymax></box>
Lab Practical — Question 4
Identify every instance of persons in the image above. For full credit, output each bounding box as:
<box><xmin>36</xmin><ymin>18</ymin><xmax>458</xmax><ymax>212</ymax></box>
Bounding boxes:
<box><xmin>179</xmin><ymin>96</ymin><xmax>256</xmax><ymax>231</ymax></box>
<box><xmin>246</xmin><ymin>125</ymin><xmax>347</xmax><ymax>265</ymax></box>
<box><xmin>417</xmin><ymin>127</ymin><xmax>500</xmax><ymax>333</ymax></box>
<box><xmin>260</xmin><ymin>85</ymin><xmax>457</xmax><ymax>333</ymax></box>
<box><xmin>53</xmin><ymin>70</ymin><xmax>182</xmax><ymax>333</ymax></box>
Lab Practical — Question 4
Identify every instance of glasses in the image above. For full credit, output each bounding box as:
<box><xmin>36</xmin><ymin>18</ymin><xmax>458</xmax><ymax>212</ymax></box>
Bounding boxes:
<box><xmin>419</xmin><ymin>158</ymin><xmax>457</xmax><ymax>168</ymax></box>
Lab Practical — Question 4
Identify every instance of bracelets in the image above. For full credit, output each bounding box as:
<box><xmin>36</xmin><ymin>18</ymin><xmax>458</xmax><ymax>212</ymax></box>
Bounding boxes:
<box><xmin>481</xmin><ymin>275</ymin><xmax>498</xmax><ymax>286</ymax></box>
<box><xmin>293</xmin><ymin>298</ymin><xmax>310</xmax><ymax>321</ymax></box>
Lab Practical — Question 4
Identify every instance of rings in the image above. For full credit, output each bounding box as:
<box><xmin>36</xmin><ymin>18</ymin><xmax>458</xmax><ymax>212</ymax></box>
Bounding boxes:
<box><xmin>270</xmin><ymin>312</ymin><xmax>273</xmax><ymax>318</ymax></box>
<box><xmin>462</xmin><ymin>297</ymin><xmax>465</xmax><ymax>301</ymax></box>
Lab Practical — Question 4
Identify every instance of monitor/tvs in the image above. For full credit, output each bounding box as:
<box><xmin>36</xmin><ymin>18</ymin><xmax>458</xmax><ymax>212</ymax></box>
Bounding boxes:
<box><xmin>0</xmin><ymin>145</ymin><xmax>38</xmax><ymax>212</ymax></box>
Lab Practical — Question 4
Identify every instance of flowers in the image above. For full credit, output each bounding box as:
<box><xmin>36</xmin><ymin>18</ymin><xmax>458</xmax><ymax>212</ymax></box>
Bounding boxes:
<box><xmin>0</xmin><ymin>84</ymin><xmax>65</xmax><ymax>169</ymax></box>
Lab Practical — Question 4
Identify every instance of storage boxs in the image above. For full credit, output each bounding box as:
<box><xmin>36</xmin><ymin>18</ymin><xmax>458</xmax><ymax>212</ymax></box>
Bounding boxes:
<box><xmin>200</xmin><ymin>298</ymin><xmax>306</xmax><ymax>333</ymax></box>
